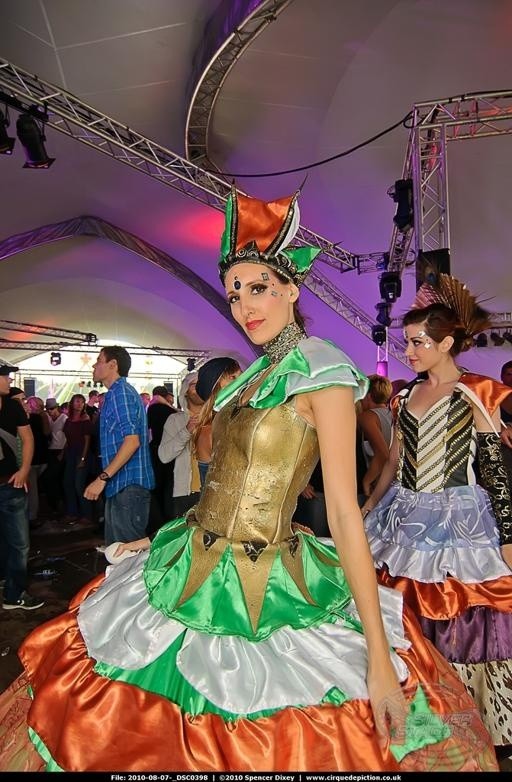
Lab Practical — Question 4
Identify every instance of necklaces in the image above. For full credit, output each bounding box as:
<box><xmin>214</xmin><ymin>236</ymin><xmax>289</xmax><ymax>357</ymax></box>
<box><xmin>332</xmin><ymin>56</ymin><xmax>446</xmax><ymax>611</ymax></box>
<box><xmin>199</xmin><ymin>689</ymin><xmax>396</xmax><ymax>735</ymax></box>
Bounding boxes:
<box><xmin>263</xmin><ymin>320</ymin><xmax>306</xmax><ymax>362</ymax></box>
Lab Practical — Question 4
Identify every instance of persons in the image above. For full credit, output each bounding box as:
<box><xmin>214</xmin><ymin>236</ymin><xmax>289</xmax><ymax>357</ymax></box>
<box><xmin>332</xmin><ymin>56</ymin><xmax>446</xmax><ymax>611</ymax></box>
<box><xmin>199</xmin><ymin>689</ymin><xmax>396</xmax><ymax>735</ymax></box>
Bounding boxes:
<box><xmin>292</xmin><ymin>373</ymin><xmax>408</xmax><ymax>537</ymax></box>
<box><xmin>502</xmin><ymin>360</ymin><xmax>512</xmax><ymax>476</ymax></box>
<box><xmin>359</xmin><ymin>304</ymin><xmax>512</xmax><ymax>762</ymax></box>
<box><xmin>0</xmin><ymin>171</ymin><xmax>499</xmax><ymax>772</ymax></box>
<box><xmin>0</xmin><ymin>345</ymin><xmax>242</xmax><ymax>610</ymax></box>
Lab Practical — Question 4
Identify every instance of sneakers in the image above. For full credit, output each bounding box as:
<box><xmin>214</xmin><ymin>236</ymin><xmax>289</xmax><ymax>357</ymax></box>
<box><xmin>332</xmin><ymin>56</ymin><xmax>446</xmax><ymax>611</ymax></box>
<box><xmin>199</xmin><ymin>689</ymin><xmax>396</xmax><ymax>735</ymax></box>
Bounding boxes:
<box><xmin>3</xmin><ymin>591</ymin><xmax>46</xmax><ymax>611</ymax></box>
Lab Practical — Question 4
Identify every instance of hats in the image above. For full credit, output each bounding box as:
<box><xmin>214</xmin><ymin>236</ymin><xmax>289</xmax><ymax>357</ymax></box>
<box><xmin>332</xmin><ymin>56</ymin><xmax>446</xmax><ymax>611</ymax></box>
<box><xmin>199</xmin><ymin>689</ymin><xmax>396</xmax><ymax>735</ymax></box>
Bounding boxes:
<box><xmin>45</xmin><ymin>398</ymin><xmax>60</xmax><ymax>409</ymax></box>
<box><xmin>194</xmin><ymin>357</ymin><xmax>231</xmax><ymax>401</ymax></box>
<box><xmin>0</xmin><ymin>359</ymin><xmax>19</xmax><ymax>375</ymax></box>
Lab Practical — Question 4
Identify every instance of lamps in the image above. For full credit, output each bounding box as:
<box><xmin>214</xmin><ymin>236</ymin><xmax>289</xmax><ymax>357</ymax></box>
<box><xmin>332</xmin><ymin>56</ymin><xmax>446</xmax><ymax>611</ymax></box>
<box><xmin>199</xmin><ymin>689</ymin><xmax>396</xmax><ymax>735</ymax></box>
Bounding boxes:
<box><xmin>50</xmin><ymin>349</ymin><xmax>62</xmax><ymax>365</ymax></box>
<box><xmin>466</xmin><ymin>327</ymin><xmax>512</xmax><ymax>351</ymax></box>
<box><xmin>186</xmin><ymin>356</ymin><xmax>197</xmax><ymax>372</ymax></box>
<box><xmin>367</xmin><ymin>174</ymin><xmax>426</xmax><ymax>349</ymax></box>
<box><xmin>0</xmin><ymin>97</ymin><xmax>57</xmax><ymax>172</ymax></box>
